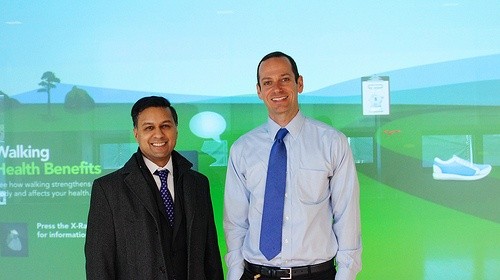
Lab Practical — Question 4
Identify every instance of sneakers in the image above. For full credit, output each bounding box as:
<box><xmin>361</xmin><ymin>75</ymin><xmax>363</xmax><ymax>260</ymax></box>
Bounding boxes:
<box><xmin>432</xmin><ymin>154</ymin><xmax>492</xmax><ymax>181</ymax></box>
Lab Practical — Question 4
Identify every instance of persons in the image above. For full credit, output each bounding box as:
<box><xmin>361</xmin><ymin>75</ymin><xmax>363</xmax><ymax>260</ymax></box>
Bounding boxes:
<box><xmin>85</xmin><ymin>96</ymin><xmax>224</xmax><ymax>280</ymax></box>
<box><xmin>224</xmin><ymin>52</ymin><xmax>361</xmax><ymax>280</ymax></box>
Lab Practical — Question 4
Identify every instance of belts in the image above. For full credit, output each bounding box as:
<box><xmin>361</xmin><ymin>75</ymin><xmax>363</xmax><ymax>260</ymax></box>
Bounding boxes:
<box><xmin>243</xmin><ymin>258</ymin><xmax>334</xmax><ymax>280</ymax></box>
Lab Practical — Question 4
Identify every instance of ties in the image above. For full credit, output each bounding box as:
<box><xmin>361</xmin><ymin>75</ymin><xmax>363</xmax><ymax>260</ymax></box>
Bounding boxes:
<box><xmin>259</xmin><ymin>128</ymin><xmax>289</xmax><ymax>262</ymax></box>
<box><xmin>153</xmin><ymin>168</ymin><xmax>175</xmax><ymax>232</ymax></box>
